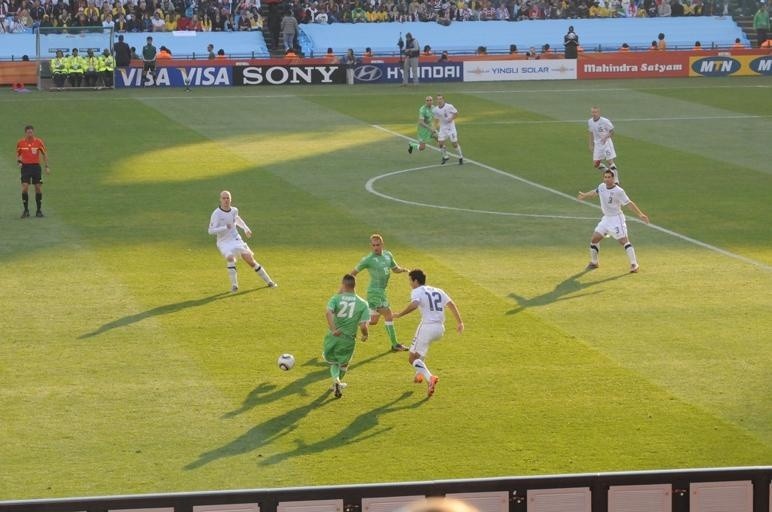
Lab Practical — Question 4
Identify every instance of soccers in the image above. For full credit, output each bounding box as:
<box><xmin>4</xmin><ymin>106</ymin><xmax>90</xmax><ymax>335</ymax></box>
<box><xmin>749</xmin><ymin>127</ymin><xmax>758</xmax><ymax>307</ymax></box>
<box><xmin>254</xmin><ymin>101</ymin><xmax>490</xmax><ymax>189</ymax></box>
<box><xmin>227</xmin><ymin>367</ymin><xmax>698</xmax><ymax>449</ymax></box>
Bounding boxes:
<box><xmin>278</xmin><ymin>353</ymin><xmax>295</xmax><ymax>370</ymax></box>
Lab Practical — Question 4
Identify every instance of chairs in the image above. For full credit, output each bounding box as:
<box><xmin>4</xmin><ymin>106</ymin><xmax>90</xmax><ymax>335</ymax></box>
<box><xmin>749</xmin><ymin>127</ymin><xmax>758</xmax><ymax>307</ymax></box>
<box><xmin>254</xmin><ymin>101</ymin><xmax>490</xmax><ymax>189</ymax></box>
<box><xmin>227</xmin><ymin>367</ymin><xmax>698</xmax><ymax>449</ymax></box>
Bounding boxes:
<box><xmin>37</xmin><ymin>60</ymin><xmax>106</xmax><ymax>86</ymax></box>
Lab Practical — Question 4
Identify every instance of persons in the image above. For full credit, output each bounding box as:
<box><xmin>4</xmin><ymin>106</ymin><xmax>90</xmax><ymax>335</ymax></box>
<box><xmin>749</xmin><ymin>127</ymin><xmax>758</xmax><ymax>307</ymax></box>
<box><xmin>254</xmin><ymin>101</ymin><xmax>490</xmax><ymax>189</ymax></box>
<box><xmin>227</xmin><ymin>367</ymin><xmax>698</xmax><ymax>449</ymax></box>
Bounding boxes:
<box><xmin>578</xmin><ymin>171</ymin><xmax>649</xmax><ymax>271</ymax></box>
<box><xmin>431</xmin><ymin>94</ymin><xmax>463</xmax><ymax>165</ymax></box>
<box><xmin>409</xmin><ymin>96</ymin><xmax>445</xmax><ymax>165</ymax></box>
<box><xmin>391</xmin><ymin>270</ymin><xmax>464</xmax><ymax>396</ymax></box>
<box><xmin>322</xmin><ymin>273</ymin><xmax>370</xmax><ymax>397</ymax></box>
<box><xmin>208</xmin><ymin>190</ymin><xmax>278</xmax><ymax>292</ymax></box>
<box><xmin>398</xmin><ymin>497</ymin><xmax>476</xmax><ymax>512</ymax></box>
<box><xmin>337</xmin><ymin>235</ymin><xmax>410</xmax><ymax>352</ymax></box>
<box><xmin>16</xmin><ymin>126</ymin><xmax>50</xmax><ymax>219</ymax></box>
<box><xmin>51</xmin><ymin>48</ymin><xmax>116</xmax><ymax>87</ymax></box>
<box><xmin>0</xmin><ymin>1</ymin><xmax>772</xmax><ymax>72</ymax></box>
<box><xmin>402</xmin><ymin>32</ymin><xmax>420</xmax><ymax>86</ymax></box>
<box><xmin>587</xmin><ymin>106</ymin><xmax>619</xmax><ymax>185</ymax></box>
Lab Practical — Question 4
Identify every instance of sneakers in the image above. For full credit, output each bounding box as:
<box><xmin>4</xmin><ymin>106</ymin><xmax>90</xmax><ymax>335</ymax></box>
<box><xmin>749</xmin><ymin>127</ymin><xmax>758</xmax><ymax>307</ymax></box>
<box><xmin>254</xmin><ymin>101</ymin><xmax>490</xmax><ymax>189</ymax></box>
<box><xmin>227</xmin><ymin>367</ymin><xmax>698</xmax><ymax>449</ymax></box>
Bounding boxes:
<box><xmin>428</xmin><ymin>376</ymin><xmax>439</xmax><ymax>397</ymax></box>
<box><xmin>459</xmin><ymin>158</ymin><xmax>462</xmax><ymax>164</ymax></box>
<box><xmin>269</xmin><ymin>281</ymin><xmax>277</xmax><ymax>288</ymax></box>
<box><xmin>588</xmin><ymin>262</ymin><xmax>599</xmax><ymax>268</ymax></box>
<box><xmin>630</xmin><ymin>265</ymin><xmax>639</xmax><ymax>273</ymax></box>
<box><xmin>440</xmin><ymin>156</ymin><xmax>450</xmax><ymax>165</ymax></box>
<box><xmin>22</xmin><ymin>211</ymin><xmax>29</xmax><ymax>218</ymax></box>
<box><xmin>415</xmin><ymin>375</ymin><xmax>423</xmax><ymax>383</ymax></box>
<box><xmin>329</xmin><ymin>382</ymin><xmax>347</xmax><ymax>397</ymax></box>
<box><xmin>232</xmin><ymin>285</ymin><xmax>238</xmax><ymax>292</ymax></box>
<box><xmin>36</xmin><ymin>212</ymin><xmax>42</xmax><ymax>216</ymax></box>
<box><xmin>392</xmin><ymin>343</ymin><xmax>409</xmax><ymax>351</ymax></box>
<box><xmin>408</xmin><ymin>142</ymin><xmax>412</xmax><ymax>153</ymax></box>
